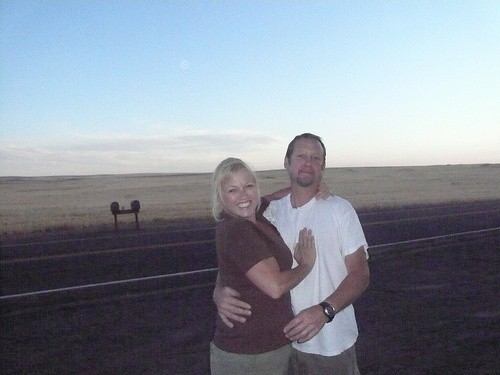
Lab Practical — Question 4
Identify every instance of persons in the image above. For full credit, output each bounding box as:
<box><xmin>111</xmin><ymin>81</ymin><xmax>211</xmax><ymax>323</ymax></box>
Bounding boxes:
<box><xmin>213</xmin><ymin>132</ymin><xmax>371</xmax><ymax>375</ymax></box>
<box><xmin>210</xmin><ymin>157</ymin><xmax>317</xmax><ymax>374</ymax></box>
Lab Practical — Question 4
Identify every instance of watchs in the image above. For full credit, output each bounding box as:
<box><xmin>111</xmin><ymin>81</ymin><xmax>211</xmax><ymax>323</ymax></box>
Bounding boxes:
<box><xmin>318</xmin><ymin>302</ymin><xmax>336</xmax><ymax>323</ymax></box>
